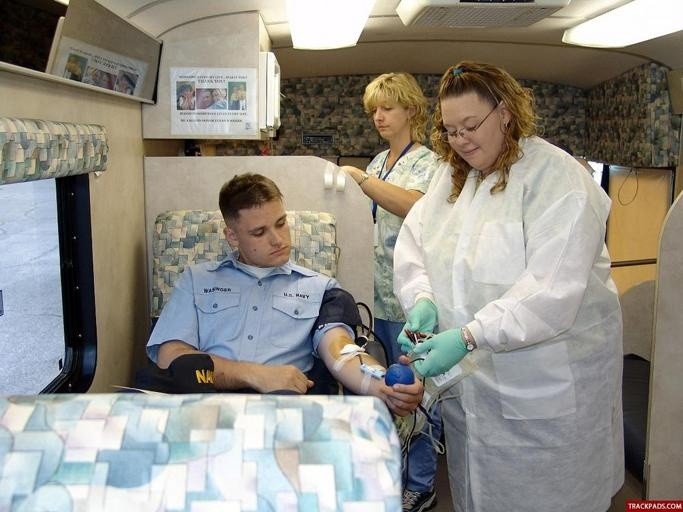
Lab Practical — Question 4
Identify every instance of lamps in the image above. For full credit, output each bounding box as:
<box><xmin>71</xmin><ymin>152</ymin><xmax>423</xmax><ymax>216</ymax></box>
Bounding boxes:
<box><xmin>562</xmin><ymin>0</ymin><xmax>683</xmax><ymax>48</ymax></box>
<box><xmin>285</xmin><ymin>0</ymin><xmax>372</xmax><ymax>50</ymax></box>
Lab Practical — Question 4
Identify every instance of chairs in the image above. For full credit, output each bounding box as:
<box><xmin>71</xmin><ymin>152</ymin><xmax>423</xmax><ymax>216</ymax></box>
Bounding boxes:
<box><xmin>111</xmin><ymin>209</ymin><xmax>344</xmax><ymax>395</ymax></box>
<box><xmin>608</xmin><ymin>352</ymin><xmax>650</xmax><ymax>512</ymax></box>
<box><xmin>1</xmin><ymin>393</ymin><xmax>405</xmax><ymax>512</ymax></box>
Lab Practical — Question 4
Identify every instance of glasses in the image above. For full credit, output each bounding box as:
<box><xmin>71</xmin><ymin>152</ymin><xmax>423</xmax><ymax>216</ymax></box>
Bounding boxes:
<box><xmin>440</xmin><ymin>103</ymin><xmax>498</xmax><ymax>144</ymax></box>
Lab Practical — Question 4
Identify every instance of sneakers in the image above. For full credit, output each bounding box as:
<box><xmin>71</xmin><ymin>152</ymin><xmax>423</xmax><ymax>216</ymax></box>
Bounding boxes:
<box><xmin>401</xmin><ymin>483</ymin><xmax>439</xmax><ymax>512</ymax></box>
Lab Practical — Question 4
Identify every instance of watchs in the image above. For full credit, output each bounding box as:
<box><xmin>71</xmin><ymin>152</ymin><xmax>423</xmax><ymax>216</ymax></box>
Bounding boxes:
<box><xmin>359</xmin><ymin>173</ymin><xmax>369</xmax><ymax>187</ymax></box>
<box><xmin>461</xmin><ymin>326</ymin><xmax>475</xmax><ymax>353</ymax></box>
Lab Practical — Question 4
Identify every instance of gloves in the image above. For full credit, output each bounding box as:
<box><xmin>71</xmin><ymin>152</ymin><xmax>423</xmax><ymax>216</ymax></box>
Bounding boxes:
<box><xmin>412</xmin><ymin>328</ymin><xmax>470</xmax><ymax>379</ymax></box>
<box><xmin>396</xmin><ymin>298</ymin><xmax>438</xmax><ymax>353</ymax></box>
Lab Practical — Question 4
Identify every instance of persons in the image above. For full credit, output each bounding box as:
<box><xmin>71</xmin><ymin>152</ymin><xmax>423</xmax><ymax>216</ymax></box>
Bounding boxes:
<box><xmin>392</xmin><ymin>64</ymin><xmax>626</xmax><ymax>510</ymax></box>
<box><xmin>178</xmin><ymin>82</ymin><xmax>244</xmax><ymax>112</ymax></box>
<box><xmin>145</xmin><ymin>171</ymin><xmax>425</xmax><ymax>415</ymax></box>
<box><xmin>344</xmin><ymin>69</ymin><xmax>454</xmax><ymax>512</ymax></box>
<box><xmin>65</xmin><ymin>56</ymin><xmax>134</xmax><ymax>95</ymax></box>
